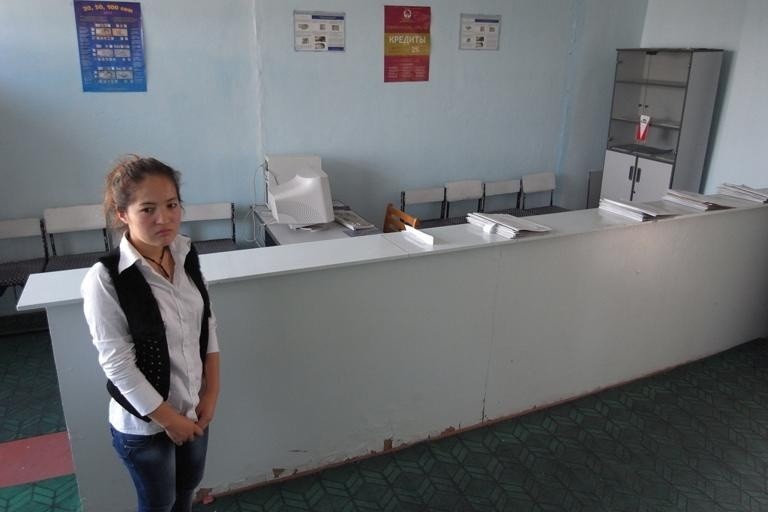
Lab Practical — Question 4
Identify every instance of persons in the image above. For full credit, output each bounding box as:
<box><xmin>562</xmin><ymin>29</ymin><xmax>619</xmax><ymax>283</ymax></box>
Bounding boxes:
<box><xmin>80</xmin><ymin>152</ymin><xmax>219</xmax><ymax>511</ymax></box>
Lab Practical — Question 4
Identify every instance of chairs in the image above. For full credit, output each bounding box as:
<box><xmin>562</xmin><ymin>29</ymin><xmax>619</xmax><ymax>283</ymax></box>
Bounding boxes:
<box><xmin>0</xmin><ymin>217</ymin><xmax>48</xmax><ymax>333</ymax></box>
<box><xmin>43</xmin><ymin>201</ymin><xmax>239</xmax><ymax>272</ymax></box>
<box><xmin>381</xmin><ymin>204</ymin><xmax>419</xmax><ymax>233</ymax></box>
<box><xmin>401</xmin><ymin>172</ymin><xmax>568</xmax><ymax>227</ymax></box>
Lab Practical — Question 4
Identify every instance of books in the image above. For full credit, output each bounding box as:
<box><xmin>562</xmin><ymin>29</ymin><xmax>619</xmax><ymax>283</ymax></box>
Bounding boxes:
<box><xmin>464</xmin><ymin>212</ymin><xmax>551</xmax><ymax>241</ymax></box>
<box><xmin>598</xmin><ymin>182</ymin><xmax>768</xmax><ymax>223</ymax></box>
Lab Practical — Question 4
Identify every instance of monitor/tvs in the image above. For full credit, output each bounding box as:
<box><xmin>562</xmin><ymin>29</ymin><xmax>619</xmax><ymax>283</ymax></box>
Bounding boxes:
<box><xmin>268</xmin><ymin>167</ymin><xmax>334</xmax><ymax>230</ymax></box>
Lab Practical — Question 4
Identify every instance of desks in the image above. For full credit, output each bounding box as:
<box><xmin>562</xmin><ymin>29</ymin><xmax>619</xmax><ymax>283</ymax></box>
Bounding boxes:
<box><xmin>251</xmin><ymin>194</ymin><xmax>377</xmax><ymax>246</ymax></box>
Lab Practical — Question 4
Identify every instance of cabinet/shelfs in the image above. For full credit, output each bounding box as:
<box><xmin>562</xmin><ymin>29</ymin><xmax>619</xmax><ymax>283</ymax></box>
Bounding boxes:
<box><xmin>599</xmin><ymin>42</ymin><xmax>726</xmax><ymax>208</ymax></box>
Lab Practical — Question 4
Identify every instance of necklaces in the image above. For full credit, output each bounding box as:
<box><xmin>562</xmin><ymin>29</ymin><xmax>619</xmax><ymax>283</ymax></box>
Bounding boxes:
<box><xmin>141</xmin><ymin>246</ymin><xmax>171</xmax><ymax>280</ymax></box>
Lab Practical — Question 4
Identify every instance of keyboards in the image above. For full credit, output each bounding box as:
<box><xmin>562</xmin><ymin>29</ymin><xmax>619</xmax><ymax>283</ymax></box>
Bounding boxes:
<box><xmin>334</xmin><ymin>211</ymin><xmax>373</xmax><ymax>230</ymax></box>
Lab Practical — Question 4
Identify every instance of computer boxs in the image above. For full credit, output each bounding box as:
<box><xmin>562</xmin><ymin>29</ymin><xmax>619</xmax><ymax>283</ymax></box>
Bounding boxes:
<box><xmin>264</xmin><ymin>153</ymin><xmax>322</xmax><ymax>212</ymax></box>
